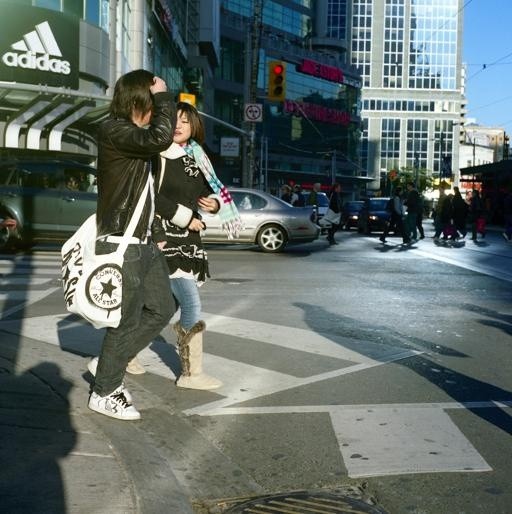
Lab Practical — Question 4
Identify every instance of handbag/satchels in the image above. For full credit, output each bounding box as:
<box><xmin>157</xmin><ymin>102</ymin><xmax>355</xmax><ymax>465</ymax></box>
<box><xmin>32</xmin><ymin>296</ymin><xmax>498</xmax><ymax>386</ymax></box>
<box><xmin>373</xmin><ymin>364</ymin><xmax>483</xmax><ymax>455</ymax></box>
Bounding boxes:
<box><xmin>323</xmin><ymin>209</ymin><xmax>342</xmax><ymax>225</ymax></box>
<box><xmin>61</xmin><ymin>213</ymin><xmax>125</xmax><ymax>330</ymax></box>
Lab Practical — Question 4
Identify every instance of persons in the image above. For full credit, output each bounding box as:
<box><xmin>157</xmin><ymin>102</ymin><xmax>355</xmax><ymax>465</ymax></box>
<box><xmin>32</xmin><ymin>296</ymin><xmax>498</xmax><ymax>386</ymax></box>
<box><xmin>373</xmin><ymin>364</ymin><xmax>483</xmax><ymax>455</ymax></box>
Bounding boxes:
<box><xmin>88</xmin><ymin>70</ymin><xmax>178</xmax><ymax>421</ymax></box>
<box><xmin>126</xmin><ymin>101</ymin><xmax>224</xmax><ymax>390</ymax></box>
<box><xmin>403</xmin><ymin>181</ymin><xmax>418</xmax><ymax>239</ymax></box>
<box><xmin>307</xmin><ymin>182</ymin><xmax>326</xmax><ymax>235</ymax></box>
<box><xmin>66</xmin><ymin>174</ymin><xmax>80</xmax><ymax>192</ymax></box>
<box><xmin>416</xmin><ymin>190</ymin><xmax>425</xmax><ymax>238</ymax></box>
<box><xmin>291</xmin><ymin>184</ymin><xmax>305</xmax><ymax>206</ymax></box>
<box><xmin>502</xmin><ymin>191</ymin><xmax>512</xmax><ymax>242</ymax></box>
<box><xmin>431</xmin><ymin>186</ymin><xmax>487</xmax><ymax>240</ymax></box>
<box><xmin>282</xmin><ymin>184</ymin><xmax>293</xmax><ymax>202</ymax></box>
<box><xmin>326</xmin><ymin>183</ymin><xmax>343</xmax><ymax>244</ymax></box>
<box><xmin>380</xmin><ymin>186</ymin><xmax>413</xmax><ymax>244</ymax></box>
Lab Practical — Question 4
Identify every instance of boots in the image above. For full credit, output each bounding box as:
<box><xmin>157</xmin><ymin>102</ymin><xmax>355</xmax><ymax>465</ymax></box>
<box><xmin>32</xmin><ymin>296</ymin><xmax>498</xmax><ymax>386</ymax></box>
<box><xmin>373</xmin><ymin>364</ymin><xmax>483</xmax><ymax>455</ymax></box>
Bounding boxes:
<box><xmin>174</xmin><ymin>319</ymin><xmax>221</xmax><ymax>390</ymax></box>
<box><xmin>125</xmin><ymin>358</ymin><xmax>145</xmax><ymax>375</ymax></box>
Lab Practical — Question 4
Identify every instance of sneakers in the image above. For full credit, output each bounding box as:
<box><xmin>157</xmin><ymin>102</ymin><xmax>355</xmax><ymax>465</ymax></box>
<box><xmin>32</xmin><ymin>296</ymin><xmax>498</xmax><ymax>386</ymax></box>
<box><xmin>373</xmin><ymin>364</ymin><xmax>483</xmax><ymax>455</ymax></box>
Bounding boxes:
<box><xmin>87</xmin><ymin>355</ymin><xmax>132</xmax><ymax>402</ymax></box>
<box><xmin>88</xmin><ymin>387</ymin><xmax>140</xmax><ymax>420</ymax></box>
<box><xmin>432</xmin><ymin>232</ymin><xmax>486</xmax><ymax>241</ymax></box>
<box><xmin>325</xmin><ymin>237</ymin><xmax>337</xmax><ymax>245</ymax></box>
<box><xmin>403</xmin><ymin>234</ymin><xmax>425</xmax><ymax>244</ymax></box>
<box><xmin>380</xmin><ymin>237</ymin><xmax>387</xmax><ymax>242</ymax></box>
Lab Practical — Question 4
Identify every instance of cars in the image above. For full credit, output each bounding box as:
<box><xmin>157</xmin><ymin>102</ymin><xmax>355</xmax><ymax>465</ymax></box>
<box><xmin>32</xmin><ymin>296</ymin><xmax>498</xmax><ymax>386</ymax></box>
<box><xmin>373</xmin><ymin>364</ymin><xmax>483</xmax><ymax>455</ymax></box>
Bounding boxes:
<box><xmin>0</xmin><ymin>159</ymin><xmax>99</xmax><ymax>254</ymax></box>
<box><xmin>198</xmin><ymin>190</ymin><xmax>408</xmax><ymax>254</ymax></box>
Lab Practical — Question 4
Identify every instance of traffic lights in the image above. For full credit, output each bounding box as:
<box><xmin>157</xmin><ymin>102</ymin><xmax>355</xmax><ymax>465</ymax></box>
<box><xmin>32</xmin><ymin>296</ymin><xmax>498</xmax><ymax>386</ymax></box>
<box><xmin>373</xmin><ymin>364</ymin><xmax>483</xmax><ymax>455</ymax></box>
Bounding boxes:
<box><xmin>268</xmin><ymin>61</ymin><xmax>286</xmax><ymax>102</ymax></box>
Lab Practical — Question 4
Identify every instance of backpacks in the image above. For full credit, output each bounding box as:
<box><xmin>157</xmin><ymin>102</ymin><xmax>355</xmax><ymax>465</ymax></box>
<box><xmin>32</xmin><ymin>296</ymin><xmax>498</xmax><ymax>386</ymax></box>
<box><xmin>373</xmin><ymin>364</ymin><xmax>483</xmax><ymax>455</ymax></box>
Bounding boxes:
<box><xmin>386</xmin><ymin>198</ymin><xmax>394</xmax><ymax>211</ymax></box>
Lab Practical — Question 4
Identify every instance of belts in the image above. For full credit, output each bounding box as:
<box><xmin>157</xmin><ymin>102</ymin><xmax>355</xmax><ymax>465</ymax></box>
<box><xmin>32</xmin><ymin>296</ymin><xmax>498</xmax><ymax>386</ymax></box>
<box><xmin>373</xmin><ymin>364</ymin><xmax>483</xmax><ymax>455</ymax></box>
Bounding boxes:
<box><xmin>98</xmin><ymin>234</ymin><xmax>148</xmax><ymax>245</ymax></box>
